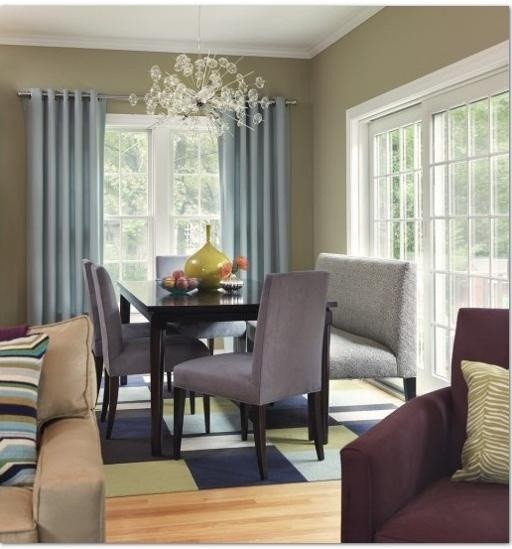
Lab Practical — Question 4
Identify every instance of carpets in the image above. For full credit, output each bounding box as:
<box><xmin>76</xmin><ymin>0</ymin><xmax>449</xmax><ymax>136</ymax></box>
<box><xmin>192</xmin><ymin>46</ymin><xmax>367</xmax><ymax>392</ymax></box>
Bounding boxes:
<box><xmin>95</xmin><ymin>369</ymin><xmax>407</xmax><ymax>497</ymax></box>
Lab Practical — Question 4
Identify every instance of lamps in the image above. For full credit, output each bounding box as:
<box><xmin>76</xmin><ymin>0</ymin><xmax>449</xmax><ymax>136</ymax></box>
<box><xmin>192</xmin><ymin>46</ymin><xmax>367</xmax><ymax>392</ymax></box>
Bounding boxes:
<box><xmin>127</xmin><ymin>5</ymin><xmax>273</xmax><ymax>145</ymax></box>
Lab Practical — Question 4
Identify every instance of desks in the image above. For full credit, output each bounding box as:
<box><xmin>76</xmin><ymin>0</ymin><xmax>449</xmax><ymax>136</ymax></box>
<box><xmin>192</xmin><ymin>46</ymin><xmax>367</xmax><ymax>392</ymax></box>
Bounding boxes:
<box><xmin>110</xmin><ymin>280</ymin><xmax>338</xmax><ymax>459</ymax></box>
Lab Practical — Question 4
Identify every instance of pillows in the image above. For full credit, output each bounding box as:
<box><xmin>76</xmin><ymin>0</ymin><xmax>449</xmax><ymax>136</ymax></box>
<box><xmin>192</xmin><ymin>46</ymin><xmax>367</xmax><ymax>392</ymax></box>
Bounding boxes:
<box><xmin>0</xmin><ymin>325</ymin><xmax>28</xmax><ymax>341</ymax></box>
<box><xmin>449</xmin><ymin>360</ymin><xmax>509</xmax><ymax>484</ymax></box>
<box><xmin>0</xmin><ymin>333</ymin><xmax>50</xmax><ymax>486</ymax></box>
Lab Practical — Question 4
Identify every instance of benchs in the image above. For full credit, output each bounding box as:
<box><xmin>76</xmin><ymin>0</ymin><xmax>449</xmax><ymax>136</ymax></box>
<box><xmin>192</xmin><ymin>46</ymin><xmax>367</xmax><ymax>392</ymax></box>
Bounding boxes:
<box><xmin>304</xmin><ymin>252</ymin><xmax>417</xmax><ymax>445</ymax></box>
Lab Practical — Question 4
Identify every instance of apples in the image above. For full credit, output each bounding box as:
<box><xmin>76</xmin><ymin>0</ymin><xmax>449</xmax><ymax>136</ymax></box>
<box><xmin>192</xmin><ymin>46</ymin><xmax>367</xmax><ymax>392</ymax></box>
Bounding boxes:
<box><xmin>162</xmin><ymin>270</ymin><xmax>199</xmax><ymax>290</ymax></box>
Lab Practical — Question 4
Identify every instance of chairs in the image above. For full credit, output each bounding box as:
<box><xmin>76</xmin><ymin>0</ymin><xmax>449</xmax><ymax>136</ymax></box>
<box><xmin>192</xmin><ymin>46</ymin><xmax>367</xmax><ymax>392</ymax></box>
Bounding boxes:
<box><xmin>341</xmin><ymin>307</ymin><xmax>509</xmax><ymax>543</ymax></box>
<box><xmin>81</xmin><ymin>258</ymin><xmax>177</xmax><ymax>405</ymax></box>
<box><xmin>173</xmin><ymin>271</ymin><xmax>328</xmax><ymax>481</ymax></box>
<box><xmin>1</xmin><ymin>315</ymin><xmax>104</xmax><ymax>543</ymax></box>
<box><xmin>91</xmin><ymin>263</ymin><xmax>210</xmax><ymax>440</ymax></box>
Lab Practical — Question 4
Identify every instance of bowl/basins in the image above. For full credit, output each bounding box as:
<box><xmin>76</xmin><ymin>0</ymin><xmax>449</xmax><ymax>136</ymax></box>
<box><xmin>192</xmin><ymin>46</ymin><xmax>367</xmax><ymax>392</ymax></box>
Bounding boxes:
<box><xmin>157</xmin><ymin>294</ymin><xmax>200</xmax><ymax>306</ymax></box>
<box><xmin>156</xmin><ymin>283</ymin><xmax>203</xmax><ymax>294</ymax></box>
